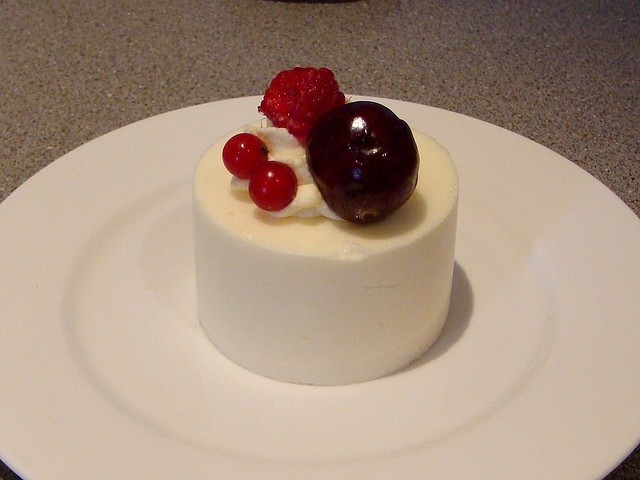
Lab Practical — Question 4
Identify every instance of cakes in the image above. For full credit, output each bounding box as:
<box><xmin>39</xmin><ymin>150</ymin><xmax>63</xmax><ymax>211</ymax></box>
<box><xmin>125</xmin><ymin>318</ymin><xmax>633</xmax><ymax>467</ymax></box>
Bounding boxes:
<box><xmin>194</xmin><ymin>67</ymin><xmax>459</xmax><ymax>387</ymax></box>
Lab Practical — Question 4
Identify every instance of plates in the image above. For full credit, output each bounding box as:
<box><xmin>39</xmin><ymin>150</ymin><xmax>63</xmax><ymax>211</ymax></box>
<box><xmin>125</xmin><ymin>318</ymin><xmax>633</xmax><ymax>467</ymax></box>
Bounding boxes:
<box><xmin>1</xmin><ymin>93</ymin><xmax>640</xmax><ymax>479</ymax></box>
